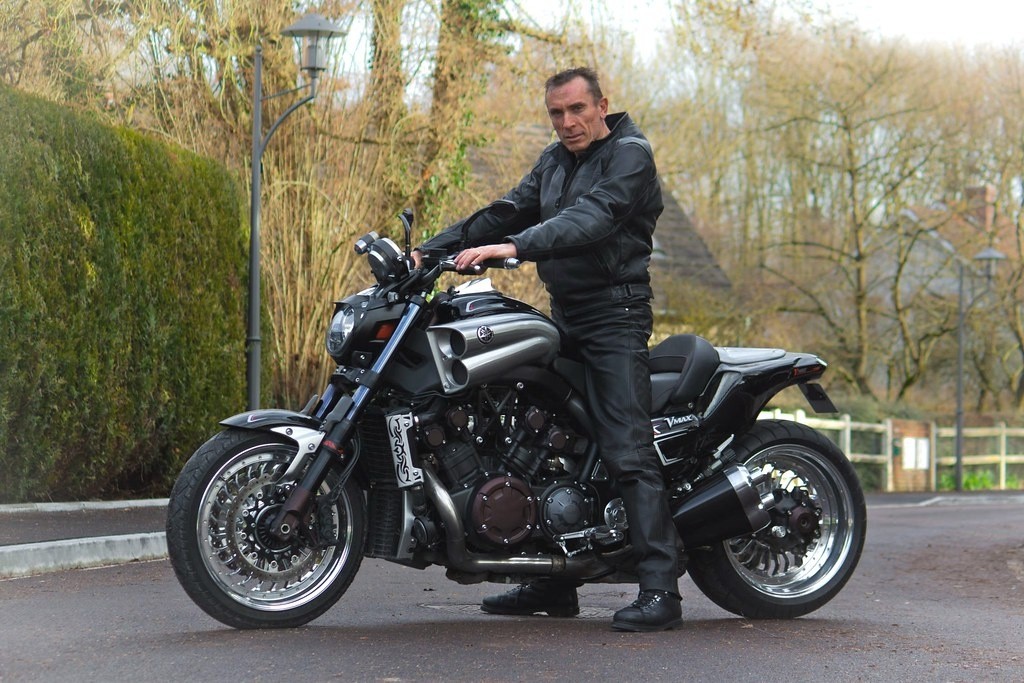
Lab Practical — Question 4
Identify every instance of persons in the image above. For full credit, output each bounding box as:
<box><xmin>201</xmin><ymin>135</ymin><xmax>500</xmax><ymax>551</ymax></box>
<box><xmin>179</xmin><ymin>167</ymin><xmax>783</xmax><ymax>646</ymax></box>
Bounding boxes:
<box><xmin>395</xmin><ymin>66</ymin><xmax>683</xmax><ymax>633</ymax></box>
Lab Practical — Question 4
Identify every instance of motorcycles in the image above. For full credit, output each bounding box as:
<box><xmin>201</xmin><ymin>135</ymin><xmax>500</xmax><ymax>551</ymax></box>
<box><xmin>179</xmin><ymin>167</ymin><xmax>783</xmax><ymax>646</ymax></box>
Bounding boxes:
<box><xmin>165</xmin><ymin>210</ymin><xmax>869</xmax><ymax>633</ymax></box>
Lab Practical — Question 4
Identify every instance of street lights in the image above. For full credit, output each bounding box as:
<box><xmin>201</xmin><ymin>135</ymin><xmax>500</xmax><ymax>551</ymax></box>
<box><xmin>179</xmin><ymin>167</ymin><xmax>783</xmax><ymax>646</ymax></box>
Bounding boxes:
<box><xmin>246</xmin><ymin>11</ymin><xmax>349</xmax><ymax>409</ymax></box>
<box><xmin>954</xmin><ymin>248</ymin><xmax>1006</xmax><ymax>492</ymax></box>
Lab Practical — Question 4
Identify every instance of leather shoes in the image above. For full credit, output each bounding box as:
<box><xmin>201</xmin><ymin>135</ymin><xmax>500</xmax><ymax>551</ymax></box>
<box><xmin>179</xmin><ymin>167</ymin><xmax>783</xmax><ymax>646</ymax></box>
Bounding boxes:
<box><xmin>609</xmin><ymin>589</ymin><xmax>684</xmax><ymax>632</ymax></box>
<box><xmin>480</xmin><ymin>584</ymin><xmax>581</xmax><ymax>618</ymax></box>
